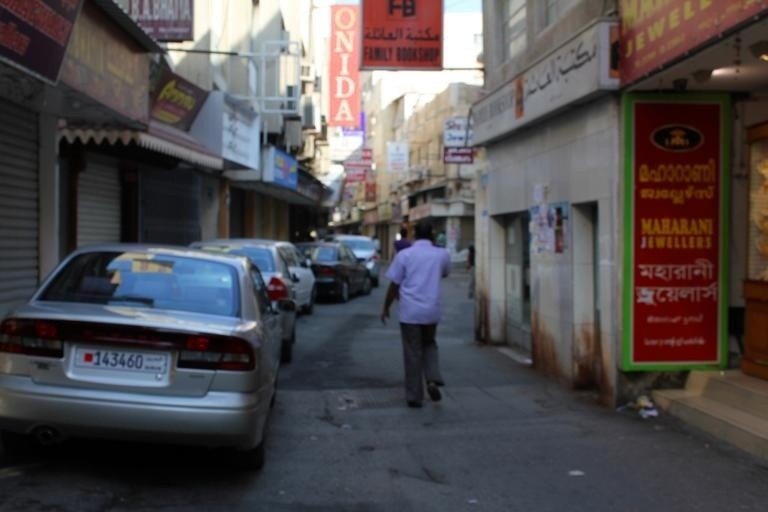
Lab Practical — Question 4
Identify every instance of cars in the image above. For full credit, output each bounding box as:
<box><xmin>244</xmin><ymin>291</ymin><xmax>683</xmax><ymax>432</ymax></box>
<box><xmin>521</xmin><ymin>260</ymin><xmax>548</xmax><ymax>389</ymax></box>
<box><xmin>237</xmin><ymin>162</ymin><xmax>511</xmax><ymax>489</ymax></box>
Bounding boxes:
<box><xmin>321</xmin><ymin>233</ymin><xmax>381</xmax><ymax>290</ymax></box>
<box><xmin>0</xmin><ymin>242</ymin><xmax>296</xmax><ymax>471</ymax></box>
<box><xmin>213</xmin><ymin>238</ymin><xmax>318</xmax><ymax>317</ymax></box>
<box><xmin>186</xmin><ymin>242</ymin><xmax>300</xmax><ymax>364</ymax></box>
<box><xmin>291</xmin><ymin>241</ymin><xmax>372</xmax><ymax>303</ymax></box>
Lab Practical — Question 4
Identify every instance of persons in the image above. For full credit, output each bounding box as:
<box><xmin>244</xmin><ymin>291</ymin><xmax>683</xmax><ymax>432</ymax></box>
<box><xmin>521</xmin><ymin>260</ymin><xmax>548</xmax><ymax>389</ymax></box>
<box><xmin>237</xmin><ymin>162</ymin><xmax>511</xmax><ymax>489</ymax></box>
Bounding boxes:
<box><xmin>435</xmin><ymin>230</ymin><xmax>447</xmax><ymax>248</ymax></box>
<box><xmin>465</xmin><ymin>243</ymin><xmax>475</xmax><ymax>299</ymax></box>
<box><xmin>393</xmin><ymin>228</ymin><xmax>411</xmax><ymax>252</ymax></box>
<box><xmin>381</xmin><ymin>221</ymin><xmax>454</xmax><ymax>407</ymax></box>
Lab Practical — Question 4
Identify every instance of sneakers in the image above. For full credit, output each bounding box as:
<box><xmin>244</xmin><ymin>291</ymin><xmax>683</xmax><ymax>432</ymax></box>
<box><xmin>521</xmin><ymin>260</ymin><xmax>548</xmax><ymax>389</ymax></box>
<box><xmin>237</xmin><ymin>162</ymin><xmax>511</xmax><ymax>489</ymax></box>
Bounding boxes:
<box><xmin>426</xmin><ymin>382</ymin><xmax>441</xmax><ymax>401</ymax></box>
